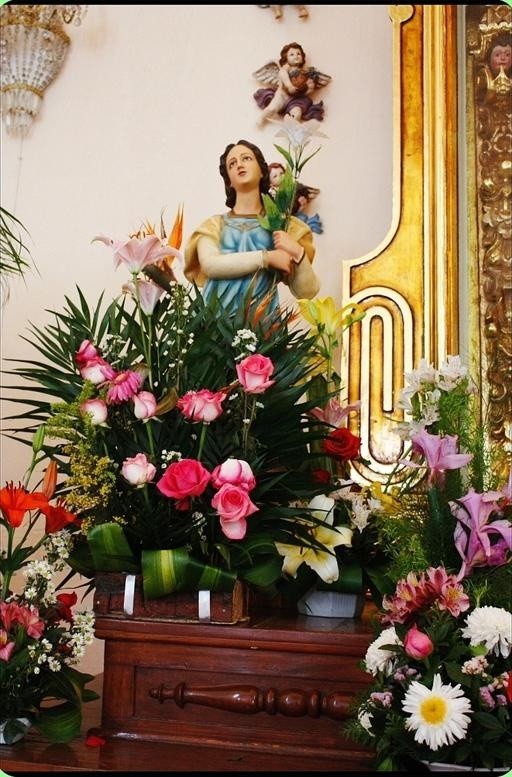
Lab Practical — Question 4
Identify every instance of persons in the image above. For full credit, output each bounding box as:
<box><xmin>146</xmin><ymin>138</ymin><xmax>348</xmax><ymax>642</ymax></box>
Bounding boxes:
<box><xmin>180</xmin><ymin>138</ymin><xmax>323</xmax><ymax>350</ymax></box>
<box><xmin>267</xmin><ymin>160</ymin><xmax>309</xmax><ymax>215</ymax></box>
<box><xmin>252</xmin><ymin>40</ymin><xmax>331</xmax><ymax>133</ymax></box>
<box><xmin>258</xmin><ymin>3</ymin><xmax>310</xmax><ymax>20</ymax></box>
<box><xmin>483</xmin><ymin>29</ymin><xmax>512</xmax><ymax>75</ymax></box>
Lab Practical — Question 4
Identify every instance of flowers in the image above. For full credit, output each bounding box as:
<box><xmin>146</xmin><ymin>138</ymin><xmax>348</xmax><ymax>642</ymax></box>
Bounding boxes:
<box><xmin>240</xmin><ymin>113</ymin><xmax>328</xmax><ymax>318</ymax></box>
<box><xmin>1</xmin><ymin>457</ymin><xmax>107</xmax><ymax>698</ymax></box>
<box><xmin>28</xmin><ymin>234</ymin><xmax>366</xmax><ymax>584</ymax></box>
<box><xmin>354</xmin><ymin>348</ymin><xmax>510</xmax><ymax>777</ymax></box>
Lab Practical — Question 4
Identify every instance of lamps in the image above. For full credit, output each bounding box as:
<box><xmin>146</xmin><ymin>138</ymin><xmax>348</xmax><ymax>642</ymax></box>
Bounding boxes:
<box><xmin>0</xmin><ymin>4</ymin><xmax>87</xmax><ymax>139</ymax></box>
